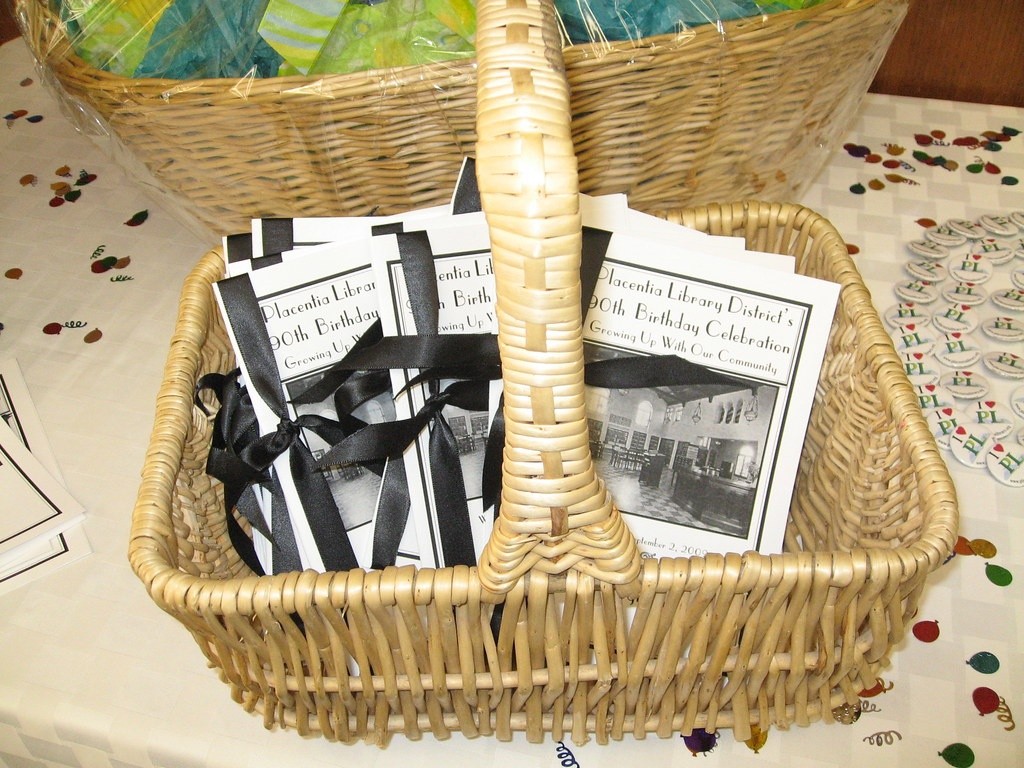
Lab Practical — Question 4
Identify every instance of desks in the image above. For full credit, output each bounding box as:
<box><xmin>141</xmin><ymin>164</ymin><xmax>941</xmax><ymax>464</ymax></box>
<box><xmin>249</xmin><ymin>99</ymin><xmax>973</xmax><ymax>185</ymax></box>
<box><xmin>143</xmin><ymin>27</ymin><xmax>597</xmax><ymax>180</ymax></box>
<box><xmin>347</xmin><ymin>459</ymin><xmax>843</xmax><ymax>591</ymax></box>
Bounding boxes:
<box><xmin>0</xmin><ymin>35</ymin><xmax>1024</xmax><ymax>768</ymax></box>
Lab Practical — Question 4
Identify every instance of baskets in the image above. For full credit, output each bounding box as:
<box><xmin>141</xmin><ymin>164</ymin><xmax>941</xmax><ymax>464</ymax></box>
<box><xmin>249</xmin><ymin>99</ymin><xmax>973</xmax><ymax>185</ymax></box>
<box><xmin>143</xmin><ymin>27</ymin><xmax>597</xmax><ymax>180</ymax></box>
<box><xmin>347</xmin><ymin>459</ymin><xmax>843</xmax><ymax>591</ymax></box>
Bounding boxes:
<box><xmin>127</xmin><ymin>0</ymin><xmax>960</xmax><ymax>746</ymax></box>
<box><xmin>14</xmin><ymin>0</ymin><xmax>911</xmax><ymax>244</ymax></box>
